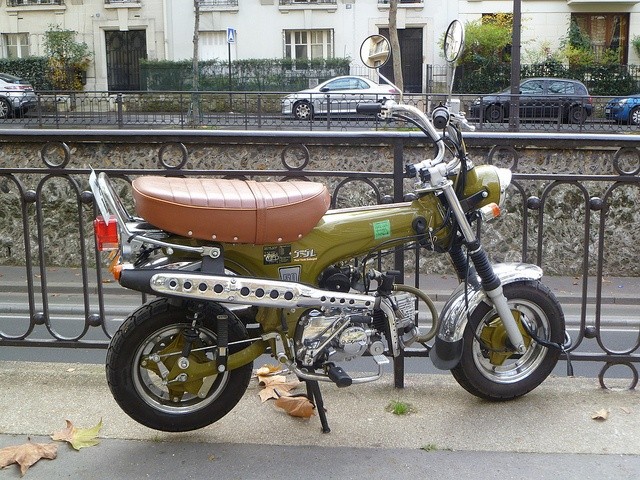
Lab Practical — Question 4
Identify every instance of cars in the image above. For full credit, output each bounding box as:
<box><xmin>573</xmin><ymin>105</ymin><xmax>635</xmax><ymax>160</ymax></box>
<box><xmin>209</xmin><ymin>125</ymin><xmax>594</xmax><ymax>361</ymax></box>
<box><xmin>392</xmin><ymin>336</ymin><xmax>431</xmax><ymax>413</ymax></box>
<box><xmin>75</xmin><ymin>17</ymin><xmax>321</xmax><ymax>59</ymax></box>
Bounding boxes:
<box><xmin>281</xmin><ymin>75</ymin><xmax>398</xmax><ymax>121</ymax></box>
<box><xmin>468</xmin><ymin>78</ymin><xmax>595</xmax><ymax>124</ymax></box>
<box><xmin>604</xmin><ymin>95</ymin><xmax>640</xmax><ymax>125</ymax></box>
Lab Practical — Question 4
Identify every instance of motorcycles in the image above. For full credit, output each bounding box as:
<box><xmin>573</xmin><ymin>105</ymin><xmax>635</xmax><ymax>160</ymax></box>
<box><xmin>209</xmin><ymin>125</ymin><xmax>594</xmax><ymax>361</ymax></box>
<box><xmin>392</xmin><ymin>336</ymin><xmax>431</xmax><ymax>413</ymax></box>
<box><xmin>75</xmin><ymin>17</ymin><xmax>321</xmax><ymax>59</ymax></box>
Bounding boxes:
<box><xmin>88</xmin><ymin>19</ymin><xmax>565</xmax><ymax>434</ymax></box>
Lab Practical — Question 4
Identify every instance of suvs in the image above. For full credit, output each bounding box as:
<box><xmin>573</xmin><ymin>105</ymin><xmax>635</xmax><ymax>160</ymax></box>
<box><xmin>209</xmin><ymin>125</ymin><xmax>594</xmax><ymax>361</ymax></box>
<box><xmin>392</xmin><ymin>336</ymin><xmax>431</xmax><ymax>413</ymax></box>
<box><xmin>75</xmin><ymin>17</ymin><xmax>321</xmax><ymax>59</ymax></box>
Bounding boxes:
<box><xmin>0</xmin><ymin>73</ymin><xmax>37</xmax><ymax>119</ymax></box>
